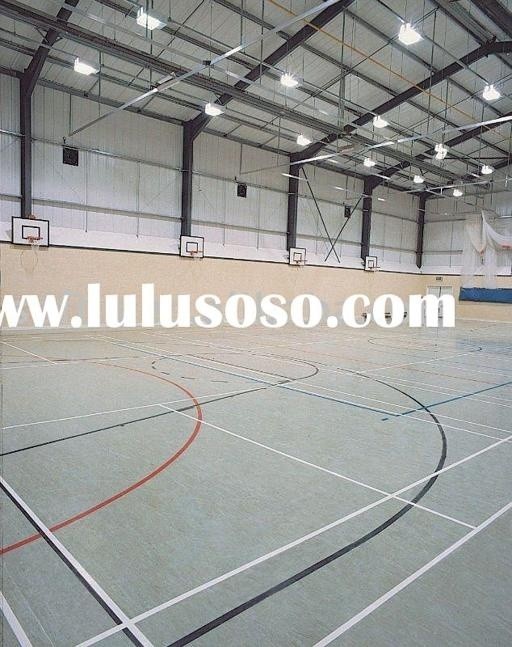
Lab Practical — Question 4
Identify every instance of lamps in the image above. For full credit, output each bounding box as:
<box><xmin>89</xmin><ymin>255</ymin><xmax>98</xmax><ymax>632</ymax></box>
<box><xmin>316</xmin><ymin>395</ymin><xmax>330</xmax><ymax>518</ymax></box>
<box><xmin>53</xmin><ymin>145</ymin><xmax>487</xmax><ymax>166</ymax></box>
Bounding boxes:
<box><xmin>413</xmin><ymin>159</ymin><xmax>426</xmax><ymax>186</ymax></box>
<box><xmin>371</xmin><ymin>95</ymin><xmax>391</xmax><ymax>131</ymax></box>
<box><xmin>71</xmin><ymin>2</ymin><xmax>101</xmax><ymax>76</ymax></box>
<box><xmin>484</xmin><ymin>62</ymin><xmax>499</xmax><ymax>102</ymax></box>
<box><xmin>281</xmin><ymin>0</ymin><xmax>301</xmax><ymax>89</ymax></box>
<box><xmin>452</xmin><ymin>184</ymin><xmax>464</xmax><ymax>195</ymax></box>
<box><xmin>397</xmin><ymin>1</ymin><xmax>424</xmax><ymax>47</ymax></box>
<box><xmin>297</xmin><ymin>92</ymin><xmax>317</xmax><ymax>149</ymax></box>
<box><xmin>363</xmin><ymin>140</ymin><xmax>378</xmax><ymax>168</ymax></box>
<box><xmin>480</xmin><ymin>144</ymin><xmax>496</xmax><ymax>177</ymax></box>
<box><xmin>435</xmin><ymin>107</ymin><xmax>450</xmax><ymax>161</ymax></box>
<box><xmin>133</xmin><ymin>1</ymin><xmax>168</xmax><ymax>32</ymax></box>
<box><xmin>203</xmin><ymin>1</ymin><xmax>228</xmax><ymax>116</ymax></box>
